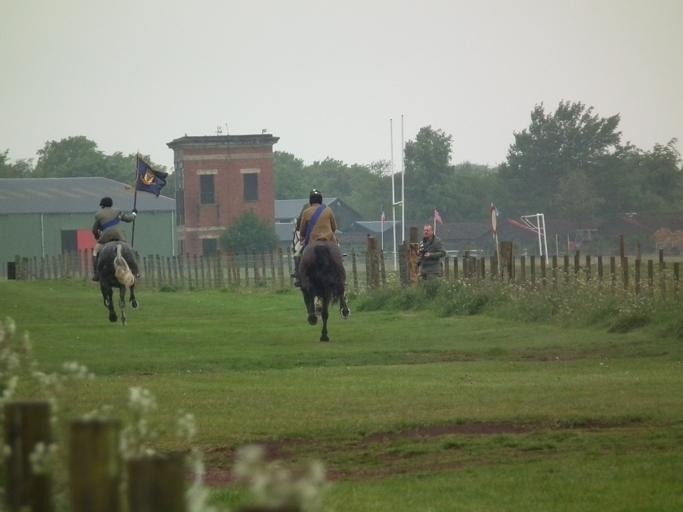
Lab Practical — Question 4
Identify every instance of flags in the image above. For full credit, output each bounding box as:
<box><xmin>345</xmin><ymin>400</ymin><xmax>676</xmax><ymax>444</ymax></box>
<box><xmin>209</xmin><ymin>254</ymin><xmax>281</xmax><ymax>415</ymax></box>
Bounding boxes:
<box><xmin>136</xmin><ymin>156</ymin><xmax>168</xmax><ymax>198</ymax></box>
<box><xmin>493</xmin><ymin>205</ymin><xmax>503</xmax><ymax>217</ymax></box>
<box><xmin>434</xmin><ymin>209</ymin><xmax>443</xmax><ymax>224</ymax></box>
<box><xmin>382</xmin><ymin>209</ymin><xmax>385</xmax><ymax>221</ymax></box>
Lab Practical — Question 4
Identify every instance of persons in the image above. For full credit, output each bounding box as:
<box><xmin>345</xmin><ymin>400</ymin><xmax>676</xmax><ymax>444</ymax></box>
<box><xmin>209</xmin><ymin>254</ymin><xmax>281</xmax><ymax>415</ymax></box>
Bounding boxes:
<box><xmin>91</xmin><ymin>197</ymin><xmax>140</xmax><ymax>281</ymax></box>
<box><xmin>293</xmin><ymin>188</ymin><xmax>337</xmax><ymax>287</ymax></box>
<box><xmin>416</xmin><ymin>224</ymin><xmax>447</xmax><ymax>280</ymax></box>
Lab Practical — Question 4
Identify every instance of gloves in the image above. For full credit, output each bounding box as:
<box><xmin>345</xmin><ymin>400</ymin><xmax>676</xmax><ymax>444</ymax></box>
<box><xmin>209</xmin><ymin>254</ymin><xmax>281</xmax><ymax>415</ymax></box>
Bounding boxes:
<box><xmin>132</xmin><ymin>209</ymin><xmax>137</xmax><ymax>216</ymax></box>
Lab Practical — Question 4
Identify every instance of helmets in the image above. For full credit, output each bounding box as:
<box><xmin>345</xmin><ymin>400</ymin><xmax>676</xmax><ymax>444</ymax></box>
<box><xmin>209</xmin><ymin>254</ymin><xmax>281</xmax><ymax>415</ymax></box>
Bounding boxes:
<box><xmin>308</xmin><ymin>189</ymin><xmax>322</xmax><ymax>203</ymax></box>
<box><xmin>99</xmin><ymin>197</ymin><xmax>112</xmax><ymax>207</ymax></box>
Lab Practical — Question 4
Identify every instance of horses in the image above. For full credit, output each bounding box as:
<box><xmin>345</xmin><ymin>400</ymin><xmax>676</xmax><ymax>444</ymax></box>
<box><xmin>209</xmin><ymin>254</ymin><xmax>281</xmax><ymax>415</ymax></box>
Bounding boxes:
<box><xmin>292</xmin><ymin>217</ymin><xmax>349</xmax><ymax>342</ymax></box>
<box><xmin>96</xmin><ymin>230</ymin><xmax>138</xmax><ymax>327</ymax></box>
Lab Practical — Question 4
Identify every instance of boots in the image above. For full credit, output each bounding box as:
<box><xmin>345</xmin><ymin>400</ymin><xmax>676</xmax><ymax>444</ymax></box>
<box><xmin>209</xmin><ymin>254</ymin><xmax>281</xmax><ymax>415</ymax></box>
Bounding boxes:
<box><xmin>291</xmin><ymin>256</ymin><xmax>302</xmax><ymax>287</ymax></box>
<box><xmin>90</xmin><ymin>255</ymin><xmax>98</xmax><ymax>282</ymax></box>
<box><xmin>124</xmin><ymin>251</ymin><xmax>138</xmax><ymax>276</ymax></box>
